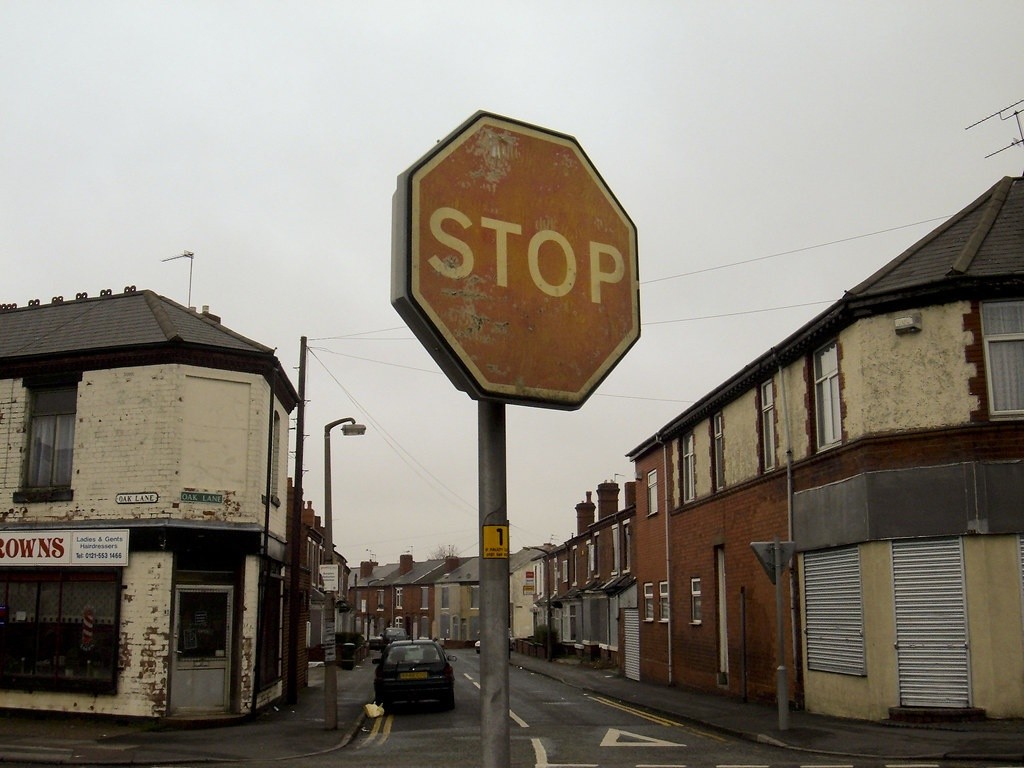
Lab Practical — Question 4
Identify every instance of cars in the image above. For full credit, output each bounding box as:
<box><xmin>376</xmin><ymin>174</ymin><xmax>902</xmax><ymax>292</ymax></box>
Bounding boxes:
<box><xmin>371</xmin><ymin>639</ymin><xmax>457</xmax><ymax>714</ymax></box>
<box><xmin>474</xmin><ymin>635</ymin><xmax>516</xmax><ymax>654</ymax></box>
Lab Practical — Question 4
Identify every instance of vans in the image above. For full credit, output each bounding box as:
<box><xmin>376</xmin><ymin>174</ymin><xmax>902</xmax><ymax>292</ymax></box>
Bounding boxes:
<box><xmin>380</xmin><ymin>628</ymin><xmax>409</xmax><ymax>649</ymax></box>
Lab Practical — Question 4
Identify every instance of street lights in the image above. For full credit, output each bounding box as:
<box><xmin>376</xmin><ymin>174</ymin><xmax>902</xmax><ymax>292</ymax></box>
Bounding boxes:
<box><xmin>522</xmin><ymin>545</ymin><xmax>552</xmax><ymax>661</ymax></box>
<box><xmin>323</xmin><ymin>417</ymin><xmax>367</xmax><ymax>731</ymax></box>
<box><xmin>365</xmin><ymin>577</ymin><xmax>386</xmax><ymax>644</ymax></box>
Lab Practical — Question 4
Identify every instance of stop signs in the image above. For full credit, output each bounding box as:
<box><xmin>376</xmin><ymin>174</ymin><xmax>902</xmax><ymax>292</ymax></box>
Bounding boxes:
<box><xmin>389</xmin><ymin>108</ymin><xmax>645</xmax><ymax>413</ymax></box>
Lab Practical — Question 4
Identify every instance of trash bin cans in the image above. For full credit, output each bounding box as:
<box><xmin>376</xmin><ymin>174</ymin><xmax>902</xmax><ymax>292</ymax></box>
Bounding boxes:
<box><xmin>342</xmin><ymin>643</ymin><xmax>355</xmax><ymax>671</ymax></box>
